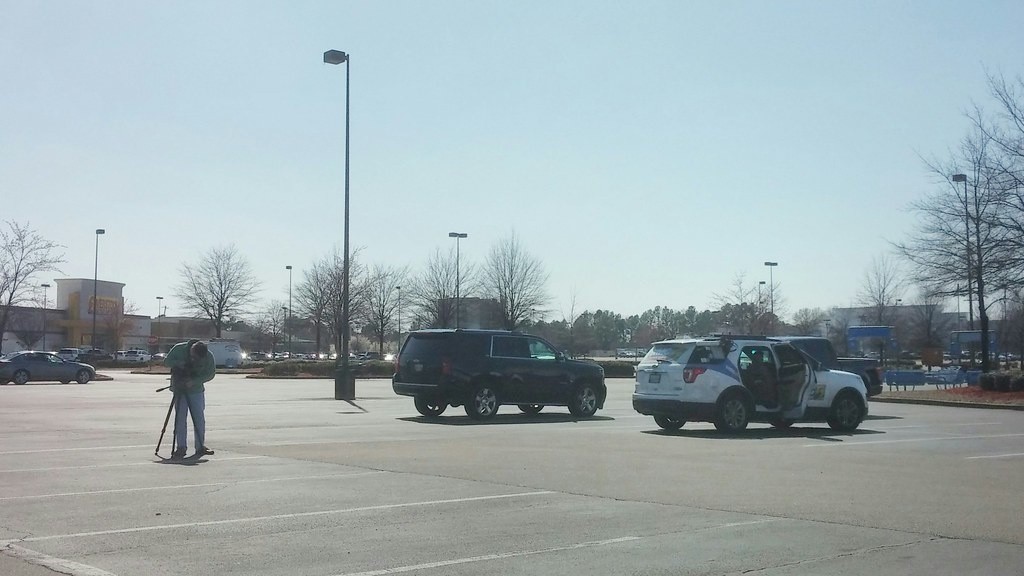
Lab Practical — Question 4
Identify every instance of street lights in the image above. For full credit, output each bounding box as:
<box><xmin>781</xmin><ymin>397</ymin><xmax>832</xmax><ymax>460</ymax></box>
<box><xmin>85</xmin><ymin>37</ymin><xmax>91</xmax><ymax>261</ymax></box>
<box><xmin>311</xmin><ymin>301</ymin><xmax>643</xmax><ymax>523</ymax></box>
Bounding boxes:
<box><xmin>324</xmin><ymin>49</ymin><xmax>355</xmax><ymax>399</ymax></box>
<box><xmin>281</xmin><ymin>307</ymin><xmax>286</xmax><ymax>344</ymax></box>
<box><xmin>449</xmin><ymin>232</ymin><xmax>468</xmax><ymax>329</ymax></box>
<box><xmin>286</xmin><ymin>265</ymin><xmax>292</xmax><ymax>360</ymax></box>
<box><xmin>896</xmin><ymin>299</ymin><xmax>902</xmax><ymax>366</ymax></box>
<box><xmin>156</xmin><ymin>296</ymin><xmax>163</xmax><ymax>352</ymax></box>
<box><xmin>531</xmin><ymin>309</ymin><xmax>535</xmax><ymax>353</ymax></box>
<box><xmin>93</xmin><ymin>228</ymin><xmax>105</xmax><ymax>350</ymax></box>
<box><xmin>396</xmin><ymin>286</ymin><xmax>400</xmax><ymax>351</ymax></box>
<box><xmin>952</xmin><ymin>174</ymin><xmax>975</xmax><ymax>358</ymax></box>
<box><xmin>758</xmin><ymin>281</ymin><xmax>766</xmax><ymax>306</ymax></box>
<box><xmin>41</xmin><ymin>284</ymin><xmax>50</xmax><ymax>351</ymax></box>
<box><xmin>766</xmin><ymin>261</ymin><xmax>778</xmax><ymax>336</ymax></box>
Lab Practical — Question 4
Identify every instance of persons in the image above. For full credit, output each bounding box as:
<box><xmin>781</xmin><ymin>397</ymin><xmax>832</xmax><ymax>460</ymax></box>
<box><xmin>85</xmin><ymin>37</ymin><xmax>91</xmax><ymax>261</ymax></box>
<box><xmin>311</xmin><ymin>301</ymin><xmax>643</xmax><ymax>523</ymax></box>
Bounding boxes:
<box><xmin>163</xmin><ymin>339</ymin><xmax>216</xmax><ymax>456</ymax></box>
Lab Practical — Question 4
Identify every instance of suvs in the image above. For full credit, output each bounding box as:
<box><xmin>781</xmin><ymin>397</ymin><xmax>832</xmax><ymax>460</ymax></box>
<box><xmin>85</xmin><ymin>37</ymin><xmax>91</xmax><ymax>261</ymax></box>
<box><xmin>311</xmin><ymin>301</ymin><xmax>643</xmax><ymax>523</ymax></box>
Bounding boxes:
<box><xmin>392</xmin><ymin>327</ymin><xmax>607</xmax><ymax>423</ymax></box>
<box><xmin>109</xmin><ymin>350</ymin><xmax>127</xmax><ymax>361</ymax></box>
<box><xmin>631</xmin><ymin>331</ymin><xmax>868</xmax><ymax>432</ymax></box>
<box><xmin>125</xmin><ymin>347</ymin><xmax>152</xmax><ymax>363</ymax></box>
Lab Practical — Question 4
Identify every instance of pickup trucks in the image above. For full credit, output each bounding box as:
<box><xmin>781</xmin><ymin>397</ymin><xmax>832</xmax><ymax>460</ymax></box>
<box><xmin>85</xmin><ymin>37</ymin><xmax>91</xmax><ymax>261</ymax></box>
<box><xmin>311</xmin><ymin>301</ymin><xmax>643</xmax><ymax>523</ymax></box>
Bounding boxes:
<box><xmin>765</xmin><ymin>336</ymin><xmax>882</xmax><ymax>399</ymax></box>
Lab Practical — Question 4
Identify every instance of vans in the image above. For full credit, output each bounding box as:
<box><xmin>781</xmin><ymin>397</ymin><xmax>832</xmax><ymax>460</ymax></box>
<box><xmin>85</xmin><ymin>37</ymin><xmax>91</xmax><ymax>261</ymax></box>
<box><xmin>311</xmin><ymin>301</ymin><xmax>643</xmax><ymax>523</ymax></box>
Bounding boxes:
<box><xmin>56</xmin><ymin>348</ymin><xmax>83</xmax><ymax>361</ymax></box>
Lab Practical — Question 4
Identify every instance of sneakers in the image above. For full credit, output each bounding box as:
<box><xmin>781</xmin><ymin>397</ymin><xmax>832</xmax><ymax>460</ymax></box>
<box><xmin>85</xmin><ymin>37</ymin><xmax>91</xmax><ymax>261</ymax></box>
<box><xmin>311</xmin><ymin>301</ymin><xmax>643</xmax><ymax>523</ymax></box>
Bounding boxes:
<box><xmin>196</xmin><ymin>449</ymin><xmax>214</xmax><ymax>455</ymax></box>
<box><xmin>176</xmin><ymin>450</ymin><xmax>186</xmax><ymax>455</ymax></box>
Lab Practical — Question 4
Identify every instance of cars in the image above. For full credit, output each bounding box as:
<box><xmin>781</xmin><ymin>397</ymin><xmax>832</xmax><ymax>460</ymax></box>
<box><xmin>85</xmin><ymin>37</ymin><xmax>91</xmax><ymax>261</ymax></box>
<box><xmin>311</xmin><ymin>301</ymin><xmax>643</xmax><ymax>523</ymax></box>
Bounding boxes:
<box><xmin>943</xmin><ymin>351</ymin><xmax>951</xmax><ymax>360</ymax></box>
<box><xmin>245</xmin><ymin>351</ymin><xmax>338</xmax><ymax>363</ymax></box>
<box><xmin>865</xmin><ymin>350</ymin><xmax>880</xmax><ymax>358</ymax></box>
<box><xmin>0</xmin><ymin>351</ymin><xmax>95</xmax><ymax>385</ymax></box>
<box><xmin>960</xmin><ymin>350</ymin><xmax>1023</xmax><ymax>361</ymax></box>
<box><xmin>152</xmin><ymin>352</ymin><xmax>167</xmax><ymax>362</ymax></box>
<box><xmin>618</xmin><ymin>351</ymin><xmax>646</xmax><ymax>358</ymax></box>
<box><xmin>348</xmin><ymin>352</ymin><xmax>381</xmax><ymax>359</ymax></box>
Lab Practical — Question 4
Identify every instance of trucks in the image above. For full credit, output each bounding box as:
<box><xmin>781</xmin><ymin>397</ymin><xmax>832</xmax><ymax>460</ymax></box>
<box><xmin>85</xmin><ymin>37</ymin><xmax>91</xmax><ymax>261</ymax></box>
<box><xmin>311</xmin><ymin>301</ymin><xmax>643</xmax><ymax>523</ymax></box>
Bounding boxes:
<box><xmin>163</xmin><ymin>338</ymin><xmax>243</xmax><ymax>369</ymax></box>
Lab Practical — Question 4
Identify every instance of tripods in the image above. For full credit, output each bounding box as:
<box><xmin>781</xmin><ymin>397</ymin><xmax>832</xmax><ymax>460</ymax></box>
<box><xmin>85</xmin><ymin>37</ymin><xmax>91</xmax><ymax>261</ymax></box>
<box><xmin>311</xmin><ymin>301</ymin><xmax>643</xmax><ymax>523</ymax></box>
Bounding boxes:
<box><xmin>154</xmin><ymin>385</ymin><xmax>187</xmax><ymax>459</ymax></box>
